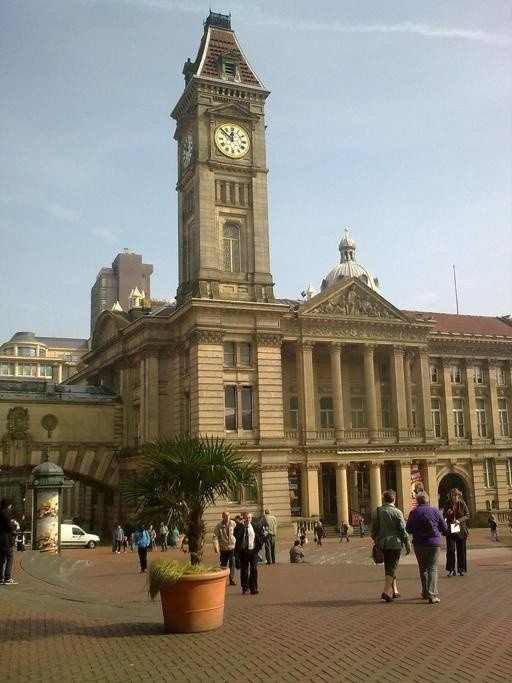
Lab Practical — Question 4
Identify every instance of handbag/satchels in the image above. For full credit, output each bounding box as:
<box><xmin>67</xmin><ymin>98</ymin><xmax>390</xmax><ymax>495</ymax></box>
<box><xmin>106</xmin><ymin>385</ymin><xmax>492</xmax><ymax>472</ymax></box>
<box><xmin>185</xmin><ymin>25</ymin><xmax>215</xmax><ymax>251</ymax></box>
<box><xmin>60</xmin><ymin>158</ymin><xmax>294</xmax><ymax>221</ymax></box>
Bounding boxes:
<box><xmin>441</xmin><ymin>518</ymin><xmax>453</xmax><ymax>537</ymax></box>
<box><xmin>371</xmin><ymin>544</ymin><xmax>385</xmax><ymax>564</ymax></box>
<box><xmin>234</xmin><ymin>556</ymin><xmax>241</xmax><ymax>570</ymax></box>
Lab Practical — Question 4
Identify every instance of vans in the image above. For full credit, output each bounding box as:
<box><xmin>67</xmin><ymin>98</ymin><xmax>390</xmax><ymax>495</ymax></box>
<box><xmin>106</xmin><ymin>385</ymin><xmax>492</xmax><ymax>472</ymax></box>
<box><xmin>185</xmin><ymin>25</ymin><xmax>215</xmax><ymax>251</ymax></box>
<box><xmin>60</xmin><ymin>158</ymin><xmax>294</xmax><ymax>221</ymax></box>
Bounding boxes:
<box><xmin>60</xmin><ymin>524</ymin><xmax>101</xmax><ymax>548</ymax></box>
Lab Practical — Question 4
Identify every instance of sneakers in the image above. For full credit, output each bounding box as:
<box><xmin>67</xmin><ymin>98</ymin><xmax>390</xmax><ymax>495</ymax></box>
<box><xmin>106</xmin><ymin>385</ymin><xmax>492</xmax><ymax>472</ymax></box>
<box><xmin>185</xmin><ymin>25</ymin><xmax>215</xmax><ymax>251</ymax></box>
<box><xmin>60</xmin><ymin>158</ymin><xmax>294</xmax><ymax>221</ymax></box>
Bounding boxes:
<box><xmin>115</xmin><ymin>550</ymin><xmax>120</xmax><ymax>554</ymax></box>
<box><xmin>0</xmin><ymin>578</ymin><xmax>5</xmax><ymax>585</ymax></box>
<box><xmin>428</xmin><ymin>597</ymin><xmax>440</xmax><ymax>604</ymax></box>
<box><xmin>421</xmin><ymin>592</ymin><xmax>428</xmax><ymax>599</ymax></box>
<box><xmin>3</xmin><ymin>579</ymin><xmax>19</xmax><ymax>585</ymax></box>
<box><xmin>392</xmin><ymin>591</ymin><xmax>402</xmax><ymax>598</ymax></box>
<box><xmin>381</xmin><ymin>591</ymin><xmax>393</xmax><ymax>602</ymax></box>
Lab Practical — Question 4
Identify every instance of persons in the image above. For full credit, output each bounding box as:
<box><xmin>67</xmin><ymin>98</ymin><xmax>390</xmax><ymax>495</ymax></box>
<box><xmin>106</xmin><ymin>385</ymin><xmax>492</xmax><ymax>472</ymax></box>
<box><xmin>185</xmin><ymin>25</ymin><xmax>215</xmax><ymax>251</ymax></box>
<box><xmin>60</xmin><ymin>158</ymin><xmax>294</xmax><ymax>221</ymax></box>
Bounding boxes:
<box><xmin>298</xmin><ymin>522</ymin><xmax>307</xmax><ymax>547</ymax></box>
<box><xmin>232</xmin><ymin>511</ymin><xmax>265</xmax><ymax>594</ymax></box>
<box><xmin>0</xmin><ymin>498</ymin><xmax>21</xmax><ymax>586</ymax></box>
<box><xmin>211</xmin><ymin>509</ymin><xmax>236</xmax><ymax>585</ymax></box>
<box><xmin>404</xmin><ymin>490</ymin><xmax>449</xmax><ymax>603</ymax></box>
<box><xmin>289</xmin><ymin>540</ymin><xmax>308</xmax><ymax>563</ymax></box>
<box><xmin>135</xmin><ymin>521</ymin><xmax>151</xmax><ymax>573</ymax></box>
<box><xmin>369</xmin><ymin>487</ymin><xmax>412</xmax><ymax>602</ymax></box>
<box><xmin>113</xmin><ymin>519</ymin><xmax>180</xmax><ymax>554</ymax></box>
<box><xmin>358</xmin><ymin>518</ymin><xmax>365</xmax><ymax>538</ymax></box>
<box><xmin>313</xmin><ymin>521</ymin><xmax>325</xmax><ymax>547</ymax></box>
<box><xmin>234</xmin><ymin>514</ymin><xmax>240</xmax><ymax>525</ymax></box>
<box><xmin>487</xmin><ymin>514</ymin><xmax>499</xmax><ymax>542</ymax></box>
<box><xmin>262</xmin><ymin>507</ymin><xmax>279</xmax><ymax>564</ymax></box>
<box><xmin>440</xmin><ymin>487</ymin><xmax>471</xmax><ymax>577</ymax></box>
<box><xmin>337</xmin><ymin>519</ymin><xmax>351</xmax><ymax>543</ymax></box>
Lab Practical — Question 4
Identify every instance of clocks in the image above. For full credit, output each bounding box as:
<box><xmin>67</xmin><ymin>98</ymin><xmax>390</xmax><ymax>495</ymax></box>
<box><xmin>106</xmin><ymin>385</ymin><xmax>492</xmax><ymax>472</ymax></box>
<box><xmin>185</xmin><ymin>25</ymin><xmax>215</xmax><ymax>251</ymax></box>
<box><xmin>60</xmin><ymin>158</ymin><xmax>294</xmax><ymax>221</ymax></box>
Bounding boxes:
<box><xmin>214</xmin><ymin>122</ymin><xmax>251</xmax><ymax>159</ymax></box>
<box><xmin>180</xmin><ymin>128</ymin><xmax>194</xmax><ymax>170</ymax></box>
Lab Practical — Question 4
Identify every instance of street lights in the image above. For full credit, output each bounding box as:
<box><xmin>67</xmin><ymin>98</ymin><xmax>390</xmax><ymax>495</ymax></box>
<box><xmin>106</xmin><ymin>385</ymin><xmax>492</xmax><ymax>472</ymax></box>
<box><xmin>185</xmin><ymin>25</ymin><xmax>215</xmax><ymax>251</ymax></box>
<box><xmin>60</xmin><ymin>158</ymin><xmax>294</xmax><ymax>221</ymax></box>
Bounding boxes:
<box><xmin>14</xmin><ymin>479</ymin><xmax>27</xmax><ymax>552</ymax></box>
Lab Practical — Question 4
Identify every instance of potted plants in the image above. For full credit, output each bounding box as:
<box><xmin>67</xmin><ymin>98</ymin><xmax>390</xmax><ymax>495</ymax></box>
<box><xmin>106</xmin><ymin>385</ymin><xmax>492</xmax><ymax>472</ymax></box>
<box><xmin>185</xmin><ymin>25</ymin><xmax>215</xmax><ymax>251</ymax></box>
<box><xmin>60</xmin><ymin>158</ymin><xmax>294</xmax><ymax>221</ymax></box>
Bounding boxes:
<box><xmin>114</xmin><ymin>431</ymin><xmax>266</xmax><ymax>634</ymax></box>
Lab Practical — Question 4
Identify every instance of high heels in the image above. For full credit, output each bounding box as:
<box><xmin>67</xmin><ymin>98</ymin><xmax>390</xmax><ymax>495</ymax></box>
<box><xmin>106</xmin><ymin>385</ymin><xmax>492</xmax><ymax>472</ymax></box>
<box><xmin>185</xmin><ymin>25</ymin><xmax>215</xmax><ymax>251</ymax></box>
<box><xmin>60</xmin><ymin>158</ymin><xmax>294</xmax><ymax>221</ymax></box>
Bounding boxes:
<box><xmin>447</xmin><ymin>570</ymin><xmax>456</xmax><ymax>577</ymax></box>
<box><xmin>460</xmin><ymin>572</ymin><xmax>464</xmax><ymax>576</ymax></box>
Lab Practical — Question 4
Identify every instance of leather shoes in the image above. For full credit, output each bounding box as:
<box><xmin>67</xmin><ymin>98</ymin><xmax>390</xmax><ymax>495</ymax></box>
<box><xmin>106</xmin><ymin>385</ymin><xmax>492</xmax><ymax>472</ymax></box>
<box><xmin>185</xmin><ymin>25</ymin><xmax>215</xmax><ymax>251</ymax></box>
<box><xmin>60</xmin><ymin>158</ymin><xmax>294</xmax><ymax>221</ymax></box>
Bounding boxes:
<box><xmin>242</xmin><ymin>590</ymin><xmax>260</xmax><ymax>594</ymax></box>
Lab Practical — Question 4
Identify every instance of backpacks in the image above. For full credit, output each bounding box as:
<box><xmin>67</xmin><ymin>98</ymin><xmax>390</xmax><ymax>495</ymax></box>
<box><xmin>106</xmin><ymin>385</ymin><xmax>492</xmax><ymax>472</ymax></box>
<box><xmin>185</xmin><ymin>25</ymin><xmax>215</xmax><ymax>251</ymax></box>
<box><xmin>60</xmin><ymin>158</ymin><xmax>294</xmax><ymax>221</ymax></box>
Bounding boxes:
<box><xmin>414</xmin><ymin>508</ymin><xmax>436</xmax><ymax>541</ymax></box>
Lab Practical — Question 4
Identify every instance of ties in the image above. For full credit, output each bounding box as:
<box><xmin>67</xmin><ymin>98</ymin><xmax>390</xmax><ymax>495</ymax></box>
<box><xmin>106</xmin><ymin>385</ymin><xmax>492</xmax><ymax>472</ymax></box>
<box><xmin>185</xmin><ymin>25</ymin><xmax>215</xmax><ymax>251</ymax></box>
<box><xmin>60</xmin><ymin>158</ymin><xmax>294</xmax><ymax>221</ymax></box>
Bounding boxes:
<box><xmin>244</xmin><ymin>526</ymin><xmax>250</xmax><ymax>553</ymax></box>
